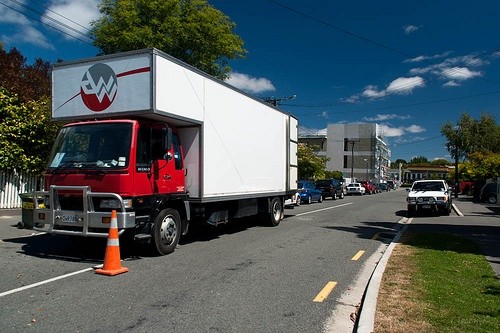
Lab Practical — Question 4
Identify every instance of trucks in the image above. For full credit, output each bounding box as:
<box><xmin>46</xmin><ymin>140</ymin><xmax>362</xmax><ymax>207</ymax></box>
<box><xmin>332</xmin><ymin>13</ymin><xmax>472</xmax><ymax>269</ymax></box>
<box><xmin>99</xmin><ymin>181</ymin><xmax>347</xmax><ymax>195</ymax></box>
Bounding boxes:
<box><xmin>32</xmin><ymin>47</ymin><xmax>298</xmax><ymax>255</ymax></box>
<box><xmin>479</xmin><ymin>177</ymin><xmax>500</xmax><ymax>204</ymax></box>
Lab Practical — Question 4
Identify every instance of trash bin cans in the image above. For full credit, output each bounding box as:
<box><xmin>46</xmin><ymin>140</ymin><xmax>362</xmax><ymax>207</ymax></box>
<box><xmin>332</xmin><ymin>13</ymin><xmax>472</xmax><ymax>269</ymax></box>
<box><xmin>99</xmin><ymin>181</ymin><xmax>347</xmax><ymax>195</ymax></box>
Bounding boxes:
<box><xmin>20</xmin><ymin>190</ymin><xmax>49</xmax><ymax>229</ymax></box>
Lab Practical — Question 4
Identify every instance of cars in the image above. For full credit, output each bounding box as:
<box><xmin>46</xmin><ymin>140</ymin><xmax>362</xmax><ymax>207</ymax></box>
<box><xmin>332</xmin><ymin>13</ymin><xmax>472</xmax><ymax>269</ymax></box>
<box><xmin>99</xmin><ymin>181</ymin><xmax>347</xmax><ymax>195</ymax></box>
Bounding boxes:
<box><xmin>283</xmin><ymin>179</ymin><xmax>323</xmax><ymax>207</ymax></box>
<box><xmin>346</xmin><ymin>183</ymin><xmax>365</xmax><ymax>195</ymax></box>
<box><xmin>361</xmin><ymin>180</ymin><xmax>397</xmax><ymax>195</ymax></box>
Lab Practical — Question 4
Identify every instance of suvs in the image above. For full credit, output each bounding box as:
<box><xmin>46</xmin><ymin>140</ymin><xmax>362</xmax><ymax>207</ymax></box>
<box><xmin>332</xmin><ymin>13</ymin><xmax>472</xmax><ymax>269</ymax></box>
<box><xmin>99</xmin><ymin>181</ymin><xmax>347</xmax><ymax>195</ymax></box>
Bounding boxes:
<box><xmin>406</xmin><ymin>179</ymin><xmax>452</xmax><ymax>215</ymax></box>
<box><xmin>315</xmin><ymin>178</ymin><xmax>344</xmax><ymax>199</ymax></box>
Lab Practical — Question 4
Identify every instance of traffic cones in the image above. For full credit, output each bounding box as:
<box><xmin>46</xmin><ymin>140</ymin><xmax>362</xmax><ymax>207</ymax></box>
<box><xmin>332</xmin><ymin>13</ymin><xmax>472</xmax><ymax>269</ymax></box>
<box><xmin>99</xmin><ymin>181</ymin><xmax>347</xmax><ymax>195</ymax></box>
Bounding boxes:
<box><xmin>95</xmin><ymin>210</ymin><xmax>129</xmax><ymax>276</ymax></box>
